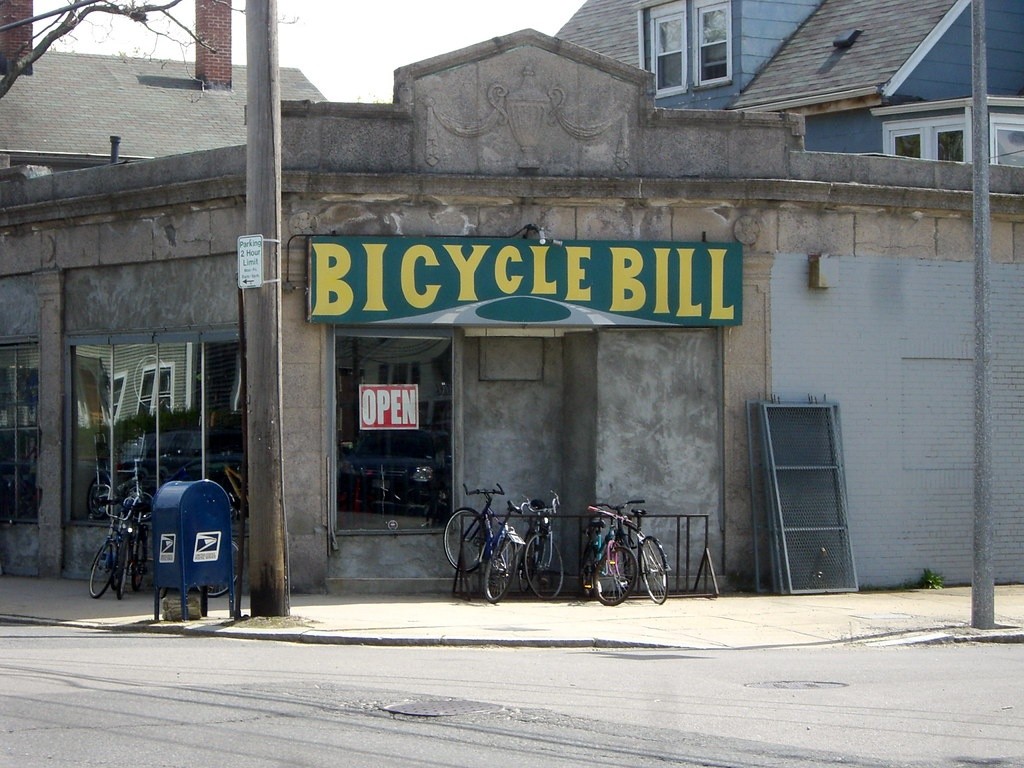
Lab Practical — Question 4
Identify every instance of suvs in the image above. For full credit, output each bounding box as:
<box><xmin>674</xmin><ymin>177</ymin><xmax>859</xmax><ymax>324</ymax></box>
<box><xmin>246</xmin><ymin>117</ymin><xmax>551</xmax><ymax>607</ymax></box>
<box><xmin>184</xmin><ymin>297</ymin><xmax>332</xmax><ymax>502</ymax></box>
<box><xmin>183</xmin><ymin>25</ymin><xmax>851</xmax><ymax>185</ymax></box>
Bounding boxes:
<box><xmin>336</xmin><ymin>426</ymin><xmax>446</xmax><ymax>515</ymax></box>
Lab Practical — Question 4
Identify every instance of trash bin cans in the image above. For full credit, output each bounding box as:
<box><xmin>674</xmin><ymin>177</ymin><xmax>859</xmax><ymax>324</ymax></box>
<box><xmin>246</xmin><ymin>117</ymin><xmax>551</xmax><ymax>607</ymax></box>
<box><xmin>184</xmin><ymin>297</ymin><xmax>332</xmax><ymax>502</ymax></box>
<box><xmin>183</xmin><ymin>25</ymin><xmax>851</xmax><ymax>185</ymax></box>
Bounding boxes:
<box><xmin>152</xmin><ymin>479</ymin><xmax>234</xmax><ymax>589</ymax></box>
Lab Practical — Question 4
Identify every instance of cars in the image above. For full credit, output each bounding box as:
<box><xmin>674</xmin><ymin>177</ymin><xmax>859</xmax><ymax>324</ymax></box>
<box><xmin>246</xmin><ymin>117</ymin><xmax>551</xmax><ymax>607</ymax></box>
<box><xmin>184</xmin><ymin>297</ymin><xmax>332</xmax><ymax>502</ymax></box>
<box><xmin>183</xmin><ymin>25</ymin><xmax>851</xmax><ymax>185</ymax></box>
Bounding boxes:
<box><xmin>164</xmin><ymin>451</ymin><xmax>248</xmax><ymax>514</ymax></box>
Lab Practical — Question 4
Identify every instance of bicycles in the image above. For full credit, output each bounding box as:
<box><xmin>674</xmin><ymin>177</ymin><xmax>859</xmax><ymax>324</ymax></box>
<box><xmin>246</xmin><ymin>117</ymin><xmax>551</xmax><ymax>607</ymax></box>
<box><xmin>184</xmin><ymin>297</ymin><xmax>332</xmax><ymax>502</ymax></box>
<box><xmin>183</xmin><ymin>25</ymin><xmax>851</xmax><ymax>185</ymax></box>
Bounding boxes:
<box><xmin>443</xmin><ymin>483</ymin><xmax>671</xmax><ymax>606</ymax></box>
<box><xmin>88</xmin><ymin>482</ymin><xmax>239</xmax><ymax>601</ymax></box>
